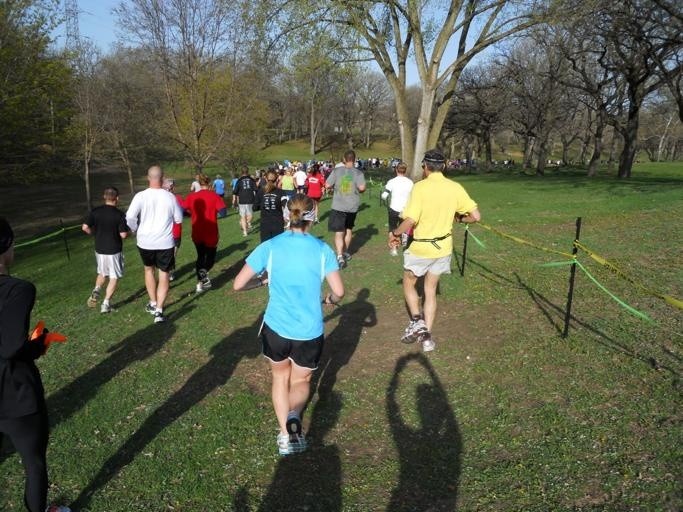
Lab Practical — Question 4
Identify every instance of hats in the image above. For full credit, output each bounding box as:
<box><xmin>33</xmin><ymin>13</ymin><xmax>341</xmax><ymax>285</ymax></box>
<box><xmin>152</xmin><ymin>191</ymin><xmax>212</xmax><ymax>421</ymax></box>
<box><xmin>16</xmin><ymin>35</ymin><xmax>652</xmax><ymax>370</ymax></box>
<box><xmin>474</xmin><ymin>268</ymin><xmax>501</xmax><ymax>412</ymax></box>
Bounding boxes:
<box><xmin>0</xmin><ymin>219</ymin><xmax>14</xmax><ymax>255</ymax></box>
<box><xmin>283</xmin><ymin>201</ymin><xmax>316</xmax><ymax>221</ymax></box>
<box><xmin>421</xmin><ymin>156</ymin><xmax>448</xmax><ymax>174</ymax></box>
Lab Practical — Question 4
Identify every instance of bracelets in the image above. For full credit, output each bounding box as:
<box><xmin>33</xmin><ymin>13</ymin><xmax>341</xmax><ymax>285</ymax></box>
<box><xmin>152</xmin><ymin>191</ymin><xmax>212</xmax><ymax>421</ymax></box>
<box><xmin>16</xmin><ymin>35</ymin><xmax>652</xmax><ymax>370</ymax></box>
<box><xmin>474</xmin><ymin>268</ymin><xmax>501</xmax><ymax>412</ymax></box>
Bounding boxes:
<box><xmin>392</xmin><ymin>229</ymin><xmax>402</xmax><ymax>237</ymax></box>
<box><xmin>328</xmin><ymin>295</ymin><xmax>338</xmax><ymax>305</ymax></box>
<box><xmin>460</xmin><ymin>215</ymin><xmax>467</xmax><ymax>223</ymax></box>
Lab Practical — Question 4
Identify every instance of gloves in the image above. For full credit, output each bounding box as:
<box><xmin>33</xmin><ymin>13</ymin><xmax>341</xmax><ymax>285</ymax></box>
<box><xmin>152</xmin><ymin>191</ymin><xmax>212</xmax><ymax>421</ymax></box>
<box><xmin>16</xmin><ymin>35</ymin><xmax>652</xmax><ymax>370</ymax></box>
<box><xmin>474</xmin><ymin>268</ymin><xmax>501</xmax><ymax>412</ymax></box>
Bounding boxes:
<box><xmin>30</xmin><ymin>328</ymin><xmax>50</xmax><ymax>358</ymax></box>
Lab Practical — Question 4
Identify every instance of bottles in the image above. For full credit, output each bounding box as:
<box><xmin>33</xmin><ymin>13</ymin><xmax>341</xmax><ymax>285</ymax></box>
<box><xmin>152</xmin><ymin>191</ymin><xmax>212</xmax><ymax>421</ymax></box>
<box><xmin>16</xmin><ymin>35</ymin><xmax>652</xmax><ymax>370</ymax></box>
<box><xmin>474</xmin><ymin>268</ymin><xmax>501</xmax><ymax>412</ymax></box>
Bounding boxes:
<box><xmin>389</xmin><ymin>231</ymin><xmax>398</xmax><ymax>256</ymax></box>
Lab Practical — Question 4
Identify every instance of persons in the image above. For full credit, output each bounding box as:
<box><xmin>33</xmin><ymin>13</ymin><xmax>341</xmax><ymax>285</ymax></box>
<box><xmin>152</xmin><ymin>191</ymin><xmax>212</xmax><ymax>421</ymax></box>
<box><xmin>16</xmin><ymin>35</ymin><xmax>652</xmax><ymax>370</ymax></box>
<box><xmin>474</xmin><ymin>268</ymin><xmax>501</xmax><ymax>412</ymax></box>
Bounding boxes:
<box><xmin>325</xmin><ymin>151</ymin><xmax>366</xmax><ymax>268</ymax></box>
<box><xmin>252</xmin><ymin>170</ymin><xmax>287</xmax><ymax>243</ymax></box>
<box><xmin>387</xmin><ymin>152</ymin><xmax>481</xmax><ymax>352</ymax></box>
<box><xmin>82</xmin><ymin>167</ymin><xmax>183</xmax><ymax>326</ymax></box>
<box><xmin>190</xmin><ymin>157</ymin><xmax>401</xmax><ymax>237</ymax></box>
<box><xmin>233</xmin><ymin>193</ymin><xmax>344</xmax><ymax>456</ymax></box>
<box><xmin>181</xmin><ymin>174</ymin><xmax>226</xmax><ymax>294</ymax></box>
<box><xmin>547</xmin><ymin>158</ymin><xmax>562</xmax><ymax>166</ymax></box>
<box><xmin>381</xmin><ymin>162</ymin><xmax>413</xmax><ymax>255</ymax></box>
<box><xmin>491</xmin><ymin>157</ymin><xmax>516</xmax><ymax>168</ymax></box>
<box><xmin>446</xmin><ymin>158</ymin><xmax>463</xmax><ymax>169</ymax></box>
<box><xmin>1</xmin><ymin>217</ymin><xmax>71</xmax><ymax>511</ymax></box>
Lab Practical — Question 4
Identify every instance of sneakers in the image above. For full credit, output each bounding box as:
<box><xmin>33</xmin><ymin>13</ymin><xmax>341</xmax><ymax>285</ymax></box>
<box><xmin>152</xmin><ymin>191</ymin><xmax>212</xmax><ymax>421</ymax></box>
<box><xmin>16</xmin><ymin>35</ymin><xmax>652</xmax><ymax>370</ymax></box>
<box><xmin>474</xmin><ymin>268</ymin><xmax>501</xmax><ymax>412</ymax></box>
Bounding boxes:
<box><xmin>337</xmin><ymin>253</ymin><xmax>351</xmax><ymax>268</ymax></box>
<box><xmin>400</xmin><ymin>318</ymin><xmax>431</xmax><ymax>344</ymax></box>
<box><xmin>146</xmin><ymin>303</ymin><xmax>164</xmax><ymax>323</ymax></box>
<box><xmin>423</xmin><ymin>338</ymin><xmax>436</xmax><ymax>353</ymax></box>
<box><xmin>101</xmin><ymin>304</ymin><xmax>119</xmax><ymax>314</ymax></box>
<box><xmin>87</xmin><ymin>289</ymin><xmax>100</xmax><ymax>307</ymax></box>
<box><xmin>242</xmin><ymin>221</ymin><xmax>253</xmax><ymax>237</ymax></box>
<box><xmin>195</xmin><ymin>272</ymin><xmax>212</xmax><ymax>292</ymax></box>
<box><xmin>276</xmin><ymin>410</ymin><xmax>307</xmax><ymax>457</ymax></box>
<box><xmin>45</xmin><ymin>505</ymin><xmax>71</xmax><ymax>512</ymax></box>
